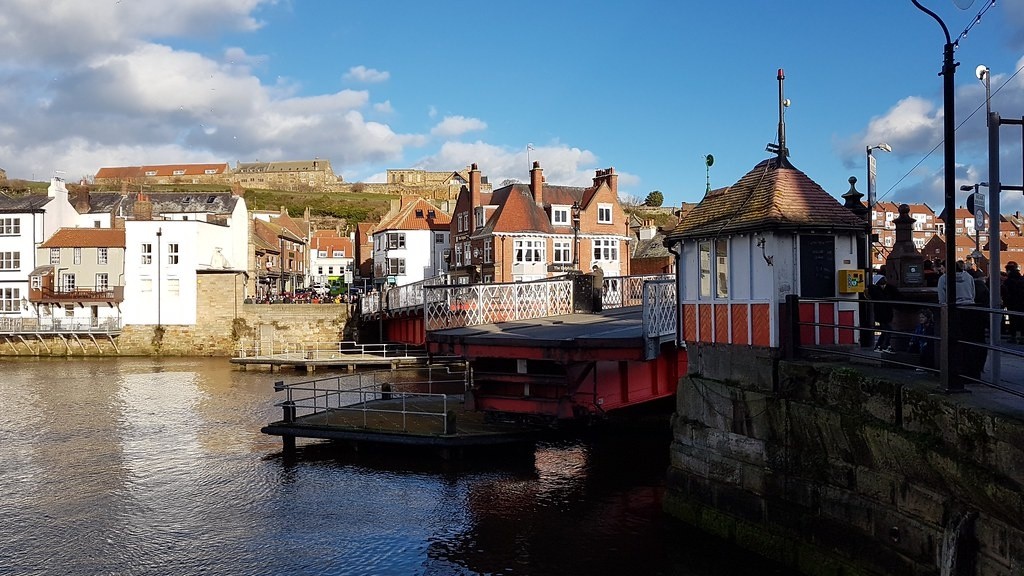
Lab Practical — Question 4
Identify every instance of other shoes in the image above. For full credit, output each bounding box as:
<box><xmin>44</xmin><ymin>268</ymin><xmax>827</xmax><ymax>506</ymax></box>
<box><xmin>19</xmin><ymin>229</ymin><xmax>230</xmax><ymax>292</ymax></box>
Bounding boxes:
<box><xmin>874</xmin><ymin>345</ymin><xmax>884</xmax><ymax>353</ymax></box>
<box><xmin>882</xmin><ymin>346</ymin><xmax>897</xmax><ymax>354</ymax></box>
<box><xmin>916</xmin><ymin>368</ymin><xmax>925</xmax><ymax>372</ymax></box>
<box><xmin>1007</xmin><ymin>337</ymin><xmax>1017</xmax><ymax>344</ymax></box>
<box><xmin>1019</xmin><ymin>337</ymin><xmax>1024</xmax><ymax>344</ymax></box>
<box><xmin>592</xmin><ymin>311</ymin><xmax>602</xmax><ymax>314</ymax></box>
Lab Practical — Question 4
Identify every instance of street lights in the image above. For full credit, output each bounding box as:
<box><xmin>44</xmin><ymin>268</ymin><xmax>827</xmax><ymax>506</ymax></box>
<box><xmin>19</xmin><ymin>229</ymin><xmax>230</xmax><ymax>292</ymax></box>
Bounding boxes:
<box><xmin>570</xmin><ymin>200</ymin><xmax>584</xmax><ymax>270</ymax></box>
<box><xmin>960</xmin><ymin>181</ymin><xmax>989</xmax><ymax>255</ymax></box>
<box><xmin>866</xmin><ymin>143</ymin><xmax>892</xmax><ymax>346</ymax></box>
<box><xmin>976</xmin><ymin>64</ymin><xmax>1001</xmax><ymax>388</ymax></box>
<box><xmin>361</xmin><ymin>278</ymin><xmax>370</xmax><ymax>295</ymax></box>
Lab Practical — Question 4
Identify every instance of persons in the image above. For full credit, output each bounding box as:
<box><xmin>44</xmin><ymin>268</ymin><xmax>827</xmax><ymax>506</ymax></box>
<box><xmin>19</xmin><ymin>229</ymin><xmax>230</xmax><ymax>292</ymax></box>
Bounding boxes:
<box><xmin>973</xmin><ymin>261</ymin><xmax>1024</xmax><ymax>383</ymax></box>
<box><xmin>938</xmin><ymin>259</ymin><xmax>977</xmax><ymax>386</ymax></box>
<box><xmin>924</xmin><ymin>259</ymin><xmax>943</xmax><ymax>287</ymax></box>
<box><xmin>872</xmin><ymin>264</ymin><xmax>888</xmax><ymax>285</ymax></box>
<box><xmin>909</xmin><ymin>310</ymin><xmax>934</xmax><ymax>371</ymax></box>
<box><xmin>872</xmin><ymin>277</ymin><xmax>900</xmax><ymax>353</ymax></box>
<box><xmin>585</xmin><ymin>265</ymin><xmax>604</xmax><ymax>313</ymax></box>
<box><xmin>957</xmin><ymin>260</ymin><xmax>975</xmax><ymax>279</ymax></box>
<box><xmin>244</xmin><ymin>289</ymin><xmax>357</xmax><ymax>304</ymax></box>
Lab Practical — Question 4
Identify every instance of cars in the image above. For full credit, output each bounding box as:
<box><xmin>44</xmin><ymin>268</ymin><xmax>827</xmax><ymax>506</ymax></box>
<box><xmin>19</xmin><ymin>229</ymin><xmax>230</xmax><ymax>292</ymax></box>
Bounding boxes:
<box><xmin>291</xmin><ymin>283</ymin><xmax>367</xmax><ymax>304</ymax></box>
